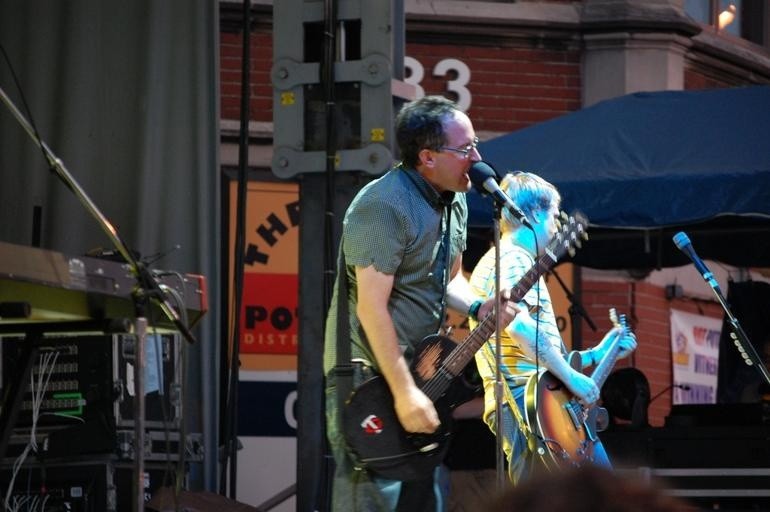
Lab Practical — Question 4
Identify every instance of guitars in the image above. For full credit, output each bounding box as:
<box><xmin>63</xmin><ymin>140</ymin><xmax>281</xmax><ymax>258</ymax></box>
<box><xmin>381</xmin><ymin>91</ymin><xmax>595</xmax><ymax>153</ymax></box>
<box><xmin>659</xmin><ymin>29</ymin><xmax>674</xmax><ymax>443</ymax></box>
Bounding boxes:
<box><xmin>523</xmin><ymin>305</ymin><xmax>633</xmax><ymax>477</ymax></box>
<box><xmin>342</xmin><ymin>209</ymin><xmax>591</xmax><ymax>482</ymax></box>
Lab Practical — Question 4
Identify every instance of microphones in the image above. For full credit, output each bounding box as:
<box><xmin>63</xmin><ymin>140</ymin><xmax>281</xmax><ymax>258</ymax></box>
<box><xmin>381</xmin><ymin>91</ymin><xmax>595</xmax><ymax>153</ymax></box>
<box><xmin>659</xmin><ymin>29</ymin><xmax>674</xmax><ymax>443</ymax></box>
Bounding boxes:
<box><xmin>649</xmin><ymin>383</ymin><xmax>691</xmax><ymax>406</ymax></box>
<box><xmin>469</xmin><ymin>162</ymin><xmax>536</xmax><ymax>233</ymax></box>
<box><xmin>673</xmin><ymin>233</ymin><xmax>722</xmax><ymax>295</ymax></box>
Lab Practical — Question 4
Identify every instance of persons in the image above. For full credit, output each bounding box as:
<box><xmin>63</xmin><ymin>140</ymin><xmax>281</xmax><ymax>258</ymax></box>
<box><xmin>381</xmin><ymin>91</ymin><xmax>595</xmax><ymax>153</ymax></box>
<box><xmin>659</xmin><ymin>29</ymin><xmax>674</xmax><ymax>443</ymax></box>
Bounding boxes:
<box><xmin>325</xmin><ymin>95</ymin><xmax>484</xmax><ymax>511</ymax></box>
<box><xmin>468</xmin><ymin>172</ymin><xmax>638</xmax><ymax>486</ymax></box>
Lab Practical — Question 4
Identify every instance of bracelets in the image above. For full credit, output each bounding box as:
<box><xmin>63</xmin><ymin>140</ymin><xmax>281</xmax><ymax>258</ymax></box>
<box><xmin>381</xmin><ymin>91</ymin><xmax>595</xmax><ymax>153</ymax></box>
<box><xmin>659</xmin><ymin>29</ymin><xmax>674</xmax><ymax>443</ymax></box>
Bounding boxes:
<box><xmin>468</xmin><ymin>298</ymin><xmax>485</xmax><ymax>322</ymax></box>
<box><xmin>586</xmin><ymin>346</ymin><xmax>597</xmax><ymax>367</ymax></box>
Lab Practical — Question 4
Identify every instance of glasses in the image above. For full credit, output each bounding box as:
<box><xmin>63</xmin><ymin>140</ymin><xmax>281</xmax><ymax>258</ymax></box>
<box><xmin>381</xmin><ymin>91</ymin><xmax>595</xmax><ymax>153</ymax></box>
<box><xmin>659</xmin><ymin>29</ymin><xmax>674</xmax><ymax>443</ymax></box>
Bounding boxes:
<box><xmin>425</xmin><ymin>136</ymin><xmax>480</xmax><ymax>159</ymax></box>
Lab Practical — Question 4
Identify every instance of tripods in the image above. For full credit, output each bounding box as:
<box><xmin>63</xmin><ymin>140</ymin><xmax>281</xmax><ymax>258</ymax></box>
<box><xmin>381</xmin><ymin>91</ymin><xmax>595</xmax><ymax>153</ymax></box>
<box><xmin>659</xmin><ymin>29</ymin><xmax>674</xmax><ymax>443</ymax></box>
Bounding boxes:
<box><xmin>1</xmin><ymin>88</ymin><xmax>196</xmax><ymax>512</ymax></box>
